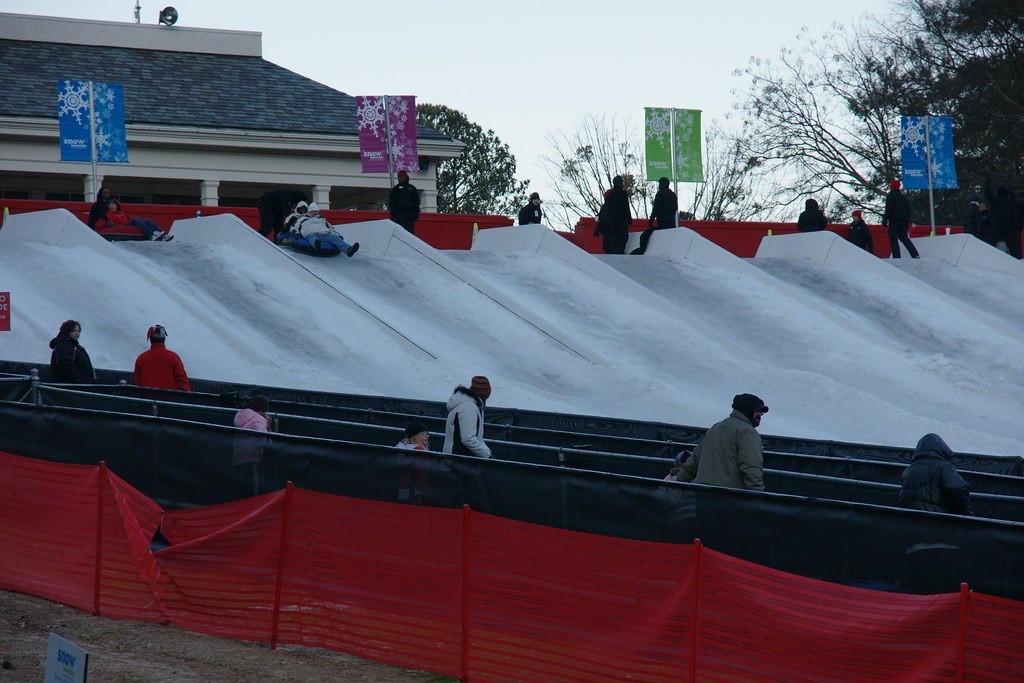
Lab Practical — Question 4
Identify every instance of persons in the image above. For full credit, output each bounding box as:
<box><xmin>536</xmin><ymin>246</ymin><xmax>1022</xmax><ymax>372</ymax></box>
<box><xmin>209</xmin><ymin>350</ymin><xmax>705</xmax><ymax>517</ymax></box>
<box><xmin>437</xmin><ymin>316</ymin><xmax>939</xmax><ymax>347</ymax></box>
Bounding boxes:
<box><xmin>443</xmin><ymin>376</ymin><xmax>493</xmax><ymax>459</ymax></box>
<box><xmin>649</xmin><ymin>176</ymin><xmax>678</xmax><ymax>228</ymax></box>
<box><xmin>274</xmin><ymin>200</ymin><xmax>359</xmax><ymax>257</ymax></box>
<box><xmin>882</xmin><ymin>180</ymin><xmax>920</xmax><ymax>259</ymax></box>
<box><xmin>234</xmin><ymin>396</ymin><xmax>272</xmax><ymax>432</ymax></box>
<box><xmin>664</xmin><ymin>393</ymin><xmax>769</xmax><ymax>491</ymax></box>
<box><xmin>963</xmin><ymin>185</ymin><xmax>1021</xmax><ymax>260</ymax></box>
<box><xmin>88</xmin><ymin>187</ymin><xmax>174</xmax><ymax>242</ymax></box>
<box><xmin>388</xmin><ymin>171</ymin><xmax>420</xmax><ymax>235</ymax></box>
<box><xmin>395</xmin><ymin>422</ymin><xmax>429</xmax><ymax>450</ymax></box>
<box><xmin>49</xmin><ymin>320</ymin><xmax>97</xmax><ymax>384</ymax></box>
<box><xmin>847</xmin><ymin>210</ymin><xmax>873</xmax><ymax>255</ymax></box>
<box><xmin>896</xmin><ymin>433</ymin><xmax>974</xmax><ymax>515</ymax></box>
<box><xmin>519</xmin><ymin>192</ymin><xmax>541</xmax><ymax>225</ymax></box>
<box><xmin>798</xmin><ymin>199</ymin><xmax>827</xmax><ymax>232</ymax></box>
<box><xmin>594</xmin><ymin>175</ymin><xmax>632</xmax><ymax>254</ymax></box>
<box><xmin>133</xmin><ymin>325</ymin><xmax>192</xmax><ymax>391</ymax></box>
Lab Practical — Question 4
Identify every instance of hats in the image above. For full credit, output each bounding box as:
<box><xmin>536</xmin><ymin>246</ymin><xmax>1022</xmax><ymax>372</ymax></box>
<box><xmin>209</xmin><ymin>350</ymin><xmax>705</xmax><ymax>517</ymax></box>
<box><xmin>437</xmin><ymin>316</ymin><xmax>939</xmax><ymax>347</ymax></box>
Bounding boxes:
<box><xmin>249</xmin><ymin>395</ymin><xmax>269</xmax><ymax>411</ymax></box>
<box><xmin>676</xmin><ymin>450</ymin><xmax>693</xmax><ymax>465</ymax></box>
<box><xmin>471</xmin><ymin>376</ymin><xmax>491</xmax><ymax>408</ymax></box>
<box><xmin>406</xmin><ymin>420</ymin><xmax>429</xmax><ymax>438</ymax></box>
<box><xmin>107</xmin><ymin>199</ymin><xmax>117</xmax><ymax>204</ymax></box>
<box><xmin>852</xmin><ymin>210</ymin><xmax>861</xmax><ymax>219</ymax></box>
<box><xmin>732</xmin><ymin>393</ymin><xmax>769</xmax><ymax>427</ymax></box>
<box><xmin>891</xmin><ymin>181</ymin><xmax>900</xmax><ymax>189</ymax></box>
<box><xmin>147</xmin><ymin>324</ymin><xmax>168</xmax><ymax>341</ymax></box>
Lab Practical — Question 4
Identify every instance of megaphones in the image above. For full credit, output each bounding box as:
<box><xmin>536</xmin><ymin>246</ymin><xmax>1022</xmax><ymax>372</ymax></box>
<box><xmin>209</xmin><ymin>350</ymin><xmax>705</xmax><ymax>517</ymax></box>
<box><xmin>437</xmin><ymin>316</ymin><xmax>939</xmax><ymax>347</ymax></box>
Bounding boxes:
<box><xmin>159</xmin><ymin>6</ymin><xmax>179</xmax><ymax>25</ymax></box>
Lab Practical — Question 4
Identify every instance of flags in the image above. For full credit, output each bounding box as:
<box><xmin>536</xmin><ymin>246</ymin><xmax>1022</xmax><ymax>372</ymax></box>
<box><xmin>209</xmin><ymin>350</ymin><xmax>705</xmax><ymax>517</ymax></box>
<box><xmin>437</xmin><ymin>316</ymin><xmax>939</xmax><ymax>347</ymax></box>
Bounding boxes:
<box><xmin>645</xmin><ymin>107</ymin><xmax>704</xmax><ymax>182</ymax></box>
<box><xmin>356</xmin><ymin>96</ymin><xmax>420</xmax><ymax>173</ymax></box>
<box><xmin>901</xmin><ymin>116</ymin><xmax>960</xmax><ymax>189</ymax></box>
<box><xmin>57</xmin><ymin>80</ymin><xmax>128</xmax><ymax>163</ymax></box>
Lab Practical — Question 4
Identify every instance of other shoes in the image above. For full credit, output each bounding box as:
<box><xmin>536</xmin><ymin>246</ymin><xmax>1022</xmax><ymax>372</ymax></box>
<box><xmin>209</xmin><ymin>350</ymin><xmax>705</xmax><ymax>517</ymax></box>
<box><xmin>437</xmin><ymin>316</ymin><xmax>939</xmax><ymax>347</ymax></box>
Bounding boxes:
<box><xmin>313</xmin><ymin>238</ymin><xmax>321</xmax><ymax>253</ymax></box>
<box><xmin>151</xmin><ymin>231</ymin><xmax>165</xmax><ymax>241</ymax></box>
<box><xmin>159</xmin><ymin>235</ymin><xmax>174</xmax><ymax>241</ymax></box>
<box><xmin>347</xmin><ymin>242</ymin><xmax>359</xmax><ymax>257</ymax></box>
